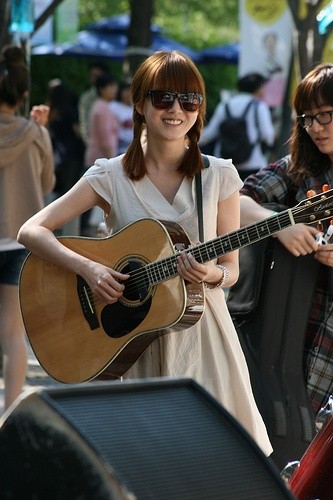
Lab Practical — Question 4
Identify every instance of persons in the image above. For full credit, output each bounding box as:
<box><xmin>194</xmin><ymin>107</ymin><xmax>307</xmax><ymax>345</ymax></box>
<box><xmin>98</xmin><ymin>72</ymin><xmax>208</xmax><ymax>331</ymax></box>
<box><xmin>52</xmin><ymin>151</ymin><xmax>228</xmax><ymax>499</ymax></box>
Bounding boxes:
<box><xmin>16</xmin><ymin>49</ymin><xmax>274</xmax><ymax>458</ymax></box>
<box><xmin>240</xmin><ymin>64</ymin><xmax>333</xmax><ymax>476</ymax></box>
<box><xmin>80</xmin><ymin>61</ymin><xmax>275</xmax><ymax>239</ymax></box>
<box><xmin>0</xmin><ymin>43</ymin><xmax>55</xmax><ymax>414</ymax></box>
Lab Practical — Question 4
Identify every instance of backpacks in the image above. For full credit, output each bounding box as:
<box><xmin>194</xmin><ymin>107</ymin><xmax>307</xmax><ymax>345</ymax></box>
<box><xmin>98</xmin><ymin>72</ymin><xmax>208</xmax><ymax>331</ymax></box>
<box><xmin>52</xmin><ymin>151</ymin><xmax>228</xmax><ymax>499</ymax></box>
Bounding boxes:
<box><xmin>218</xmin><ymin>99</ymin><xmax>261</xmax><ymax>165</ymax></box>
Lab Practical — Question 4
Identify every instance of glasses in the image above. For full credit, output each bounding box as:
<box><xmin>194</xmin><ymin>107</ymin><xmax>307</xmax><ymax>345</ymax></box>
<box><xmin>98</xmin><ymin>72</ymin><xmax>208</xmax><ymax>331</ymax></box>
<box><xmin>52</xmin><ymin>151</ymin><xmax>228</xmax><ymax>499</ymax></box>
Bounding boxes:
<box><xmin>296</xmin><ymin>111</ymin><xmax>333</xmax><ymax>128</ymax></box>
<box><xmin>146</xmin><ymin>88</ymin><xmax>203</xmax><ymax>111</ymax></box>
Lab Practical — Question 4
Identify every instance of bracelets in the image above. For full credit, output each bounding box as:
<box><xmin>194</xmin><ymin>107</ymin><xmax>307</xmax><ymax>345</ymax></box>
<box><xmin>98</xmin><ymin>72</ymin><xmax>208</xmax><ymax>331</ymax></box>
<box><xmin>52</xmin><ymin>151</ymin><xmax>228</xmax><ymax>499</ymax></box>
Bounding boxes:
<box><xmin>203</xmin><ymin>264</ymin><xmax>230</xmax><ymax>290</ymax></box>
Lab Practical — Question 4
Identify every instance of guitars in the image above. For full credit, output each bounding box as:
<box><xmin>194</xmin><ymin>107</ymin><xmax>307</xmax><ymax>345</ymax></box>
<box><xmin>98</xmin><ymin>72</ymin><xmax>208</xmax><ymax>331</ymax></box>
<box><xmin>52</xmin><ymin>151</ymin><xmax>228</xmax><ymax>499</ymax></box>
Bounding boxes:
<box><xmin>19</xmin><ymin>184</ymin><xmax>333</xmax><ymax>384</ymax></box>
